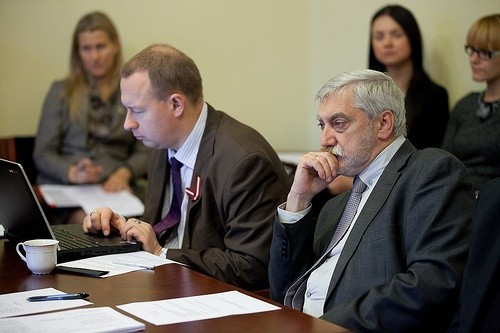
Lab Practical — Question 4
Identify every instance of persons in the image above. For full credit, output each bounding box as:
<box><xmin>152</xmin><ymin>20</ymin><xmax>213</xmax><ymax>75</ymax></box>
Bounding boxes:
<box><xmin>268</xmin><ymin>69</ymin><xmax>476</xmax><ymax>333</ymax></box>
<box><xmin>32</xmin><ymin>12</ymin><xmax>157</xmax><ymax>226</ymax></box>
<box><xmin>366</xmin><ymin>5</ymin><xmax>500</xmax><ymax>333</ymax></box>
<box><xmin>82</xmin><ymin>44</ymin><xmax>292</xmax><ymax>291</ymax></box>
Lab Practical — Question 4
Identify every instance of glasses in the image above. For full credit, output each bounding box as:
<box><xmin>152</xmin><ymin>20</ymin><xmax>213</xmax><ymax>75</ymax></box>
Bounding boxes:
<box><xmin>464</xmin><ymin>43</ymin><xmax>500</xmax><ymax>60</ymax></box>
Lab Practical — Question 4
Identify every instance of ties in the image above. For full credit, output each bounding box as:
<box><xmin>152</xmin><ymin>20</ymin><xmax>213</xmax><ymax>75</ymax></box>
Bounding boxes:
<box><xmin>152</xmin><ymin>156</ymin><xmax>185</xmax><ymax>242</ymax></box>
<box><xmin>283</xmin><ymin>176</ymin><xmax>368</xmax><ymax>312</ymax></box>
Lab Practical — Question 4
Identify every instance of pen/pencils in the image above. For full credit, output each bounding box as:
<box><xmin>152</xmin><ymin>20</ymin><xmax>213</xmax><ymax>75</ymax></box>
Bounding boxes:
<box><xmin>26</xmin><ymin>292</ymin><xmax>90</xmax><ymax>302</ymax></box>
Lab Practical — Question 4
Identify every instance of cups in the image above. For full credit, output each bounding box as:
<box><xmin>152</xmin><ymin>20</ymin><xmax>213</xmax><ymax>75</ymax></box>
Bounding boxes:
<box><xmin>15</xmin><ymin>239</ymin><xmax>59</xmax><ymax>274</ymax></box>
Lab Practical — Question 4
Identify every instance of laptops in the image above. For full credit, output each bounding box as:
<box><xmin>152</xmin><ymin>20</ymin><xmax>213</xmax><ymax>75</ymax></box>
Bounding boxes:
<box><xmin>0</xmin><ymin>158</ymin><xmax>143</xmax><ymax>261</ymax></box>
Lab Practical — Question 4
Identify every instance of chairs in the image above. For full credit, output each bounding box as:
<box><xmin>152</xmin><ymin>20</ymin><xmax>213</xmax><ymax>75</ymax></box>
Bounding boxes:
<box><xmin>7</xmin><ymin>137</ymin><xmax>43</xmax><ymax>201</ymax></box>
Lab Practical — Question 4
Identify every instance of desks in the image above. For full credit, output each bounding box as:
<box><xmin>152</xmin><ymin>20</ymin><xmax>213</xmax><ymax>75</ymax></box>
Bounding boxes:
<box><xmin>0</xmin><ymin>223</ymin><xmax>355</xmax><ymax>333</ymax></box>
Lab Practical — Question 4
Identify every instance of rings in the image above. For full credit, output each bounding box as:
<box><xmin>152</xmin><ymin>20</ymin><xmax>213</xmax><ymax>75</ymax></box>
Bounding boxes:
<box><xmin>135</xmin><ymin>219</ymin><xmax>141</xmax><ymax>225</ymax></box>
<box><xmin>89</xmin><ymin>210</ymin><xmax>99</xmax><ymax>216</ymax></box>
<box><xmin>314</xmin><ymin>155</ymin><xmax>318</xmax><ymax>159</ymax></box>
